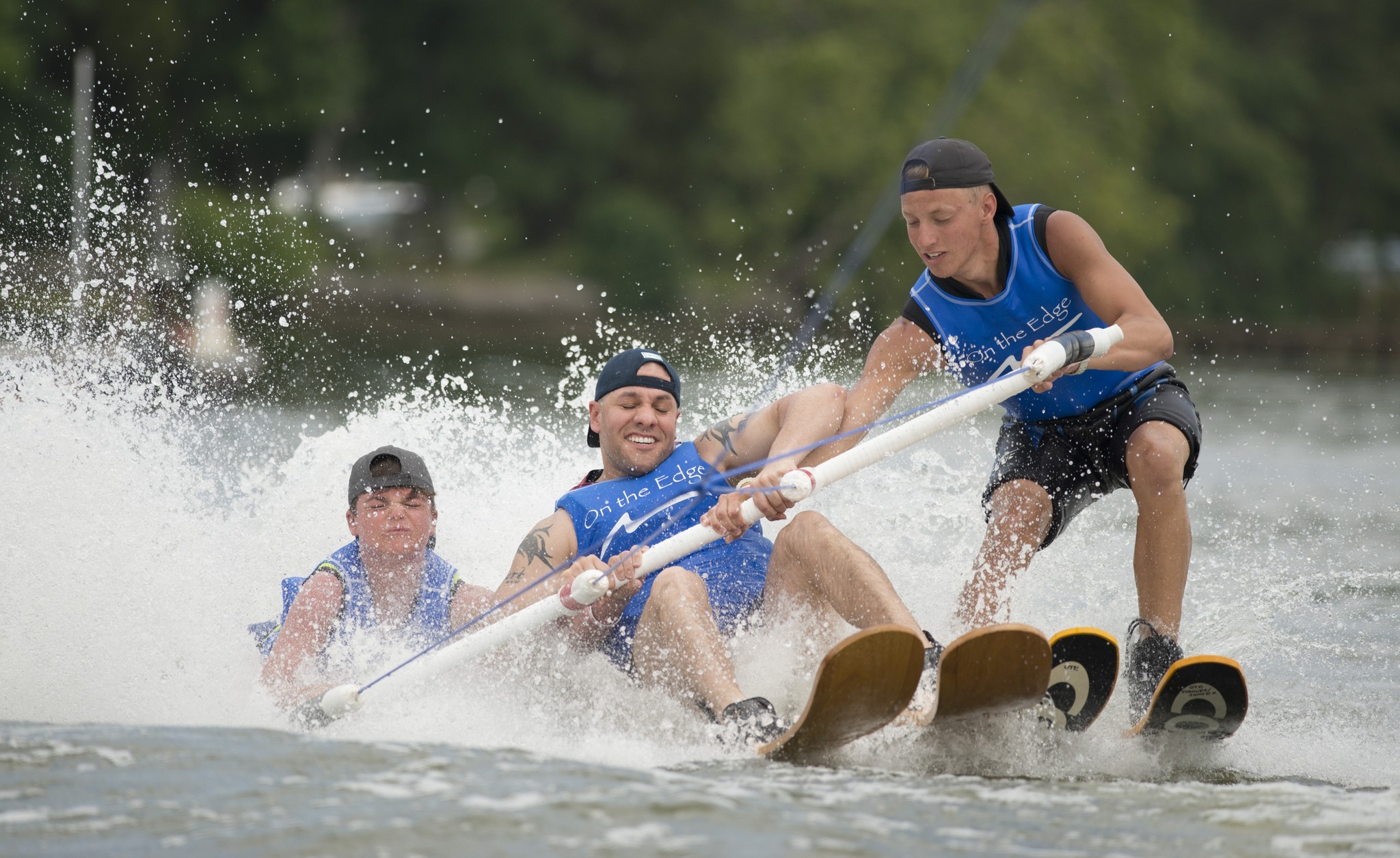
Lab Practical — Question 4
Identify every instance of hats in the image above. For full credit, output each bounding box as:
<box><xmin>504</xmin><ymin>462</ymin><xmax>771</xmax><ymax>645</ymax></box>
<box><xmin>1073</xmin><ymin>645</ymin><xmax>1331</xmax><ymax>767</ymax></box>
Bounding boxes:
<box><xmin>587</xmin><ymin>347</ymin><xmax>680</xmax><ymax>448</ymax></box>
<box><xmin>900</xmin><ymin>137</ymin><xmax>1016</xmax><ymax>217</ymax></box>
<box><xmin>348</xmin><ymin>446</ymin><xmax>435</xmax><ymax>549</ymax></box>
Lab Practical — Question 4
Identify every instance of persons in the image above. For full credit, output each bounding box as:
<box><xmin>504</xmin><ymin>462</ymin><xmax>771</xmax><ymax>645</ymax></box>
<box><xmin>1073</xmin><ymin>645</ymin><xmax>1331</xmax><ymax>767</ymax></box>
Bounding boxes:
<box><xmin>494</xmin><ymin>348</ymin><xmax>946</xmax><ymax>751</ymax></box>
<box><xmin>249</xmin><ymin>444</ymin><xmax>616</xmax><ymax>727</ymax></box>
<box><xmin>698</xmin><ymin>138</ymin><xmax>1202</xmax><ymax>726</ymax></box>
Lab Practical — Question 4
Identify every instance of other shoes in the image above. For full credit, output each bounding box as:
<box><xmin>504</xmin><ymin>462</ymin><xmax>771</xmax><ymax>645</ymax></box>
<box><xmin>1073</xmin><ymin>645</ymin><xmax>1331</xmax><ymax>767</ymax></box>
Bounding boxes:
<box><xmin>905</xmin><ymin>627</ymin><xmax>947</xmax><ymax>710</ymax></box>
<box><xmin>1122</xmin><ymin>619</ymin><xmax>1186</xmax><ymax>728</ymax></box>
<box><xmin>719</xmin><ymin>696</ymin><xmax>791</xmax><ymax>748</ymax></box>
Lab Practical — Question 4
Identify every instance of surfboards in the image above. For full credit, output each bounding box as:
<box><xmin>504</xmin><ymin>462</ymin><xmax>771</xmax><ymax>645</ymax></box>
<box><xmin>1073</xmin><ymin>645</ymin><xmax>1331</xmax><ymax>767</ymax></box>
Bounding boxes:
<box><xmin>1038</xmin><ymin>627</ymin><xmax>1120</xmax><ymax>734</ymax></box>
<box><xmin>1127</xmin><ymin>655</ymin><xmax>1249</xmax><ymax>741</ymax></box>
<box><xmin>925</xmin><ymin>623</ymin><xmax>1052</xmax><ymax>727</ymax></box>
<box><xmin>760</xmin><ymin>625</ymin><xmax>925</xmax><ymax>759</ymax></box>
